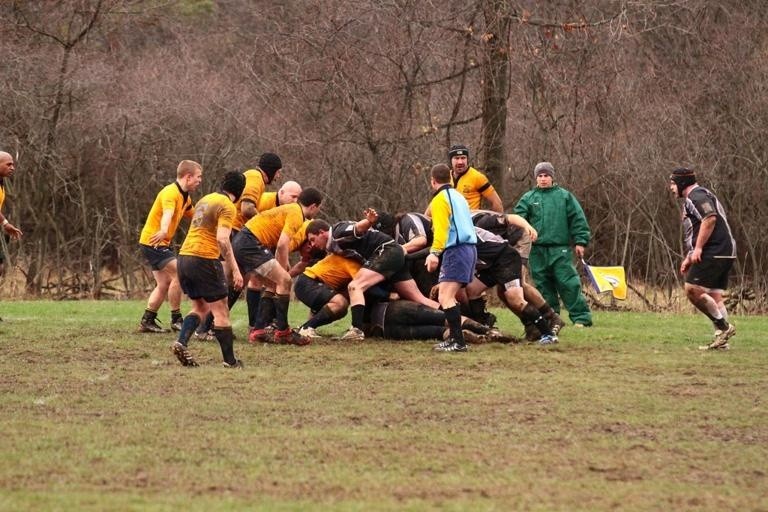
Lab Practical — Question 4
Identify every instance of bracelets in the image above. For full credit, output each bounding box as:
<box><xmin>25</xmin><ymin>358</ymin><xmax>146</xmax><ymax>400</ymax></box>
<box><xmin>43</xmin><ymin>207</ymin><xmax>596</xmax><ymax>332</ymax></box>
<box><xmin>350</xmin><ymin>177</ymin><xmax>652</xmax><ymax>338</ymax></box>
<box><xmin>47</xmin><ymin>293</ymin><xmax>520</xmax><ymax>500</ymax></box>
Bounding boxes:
<box><xmin>1</xmin><ymin>219</ymin><xmax>8</xmax><ymax>226</ymax></box>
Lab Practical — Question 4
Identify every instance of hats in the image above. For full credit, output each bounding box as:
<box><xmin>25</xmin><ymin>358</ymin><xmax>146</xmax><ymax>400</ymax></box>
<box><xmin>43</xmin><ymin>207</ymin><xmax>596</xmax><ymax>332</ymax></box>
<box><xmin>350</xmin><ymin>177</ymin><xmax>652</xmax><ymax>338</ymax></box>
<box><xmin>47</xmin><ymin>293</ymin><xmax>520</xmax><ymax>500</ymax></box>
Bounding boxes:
<box><xmin>447</xmin><ymin>144</ymin><xmax>470</xmax><ymax>157</ymax></box>
<box><xmin>257</xmin><ymin>150</ymin><xmax>284</xmax><ymax>185</ymax></box>
<box><xmin>533</xmin><ymin>162</ymin><xmax>555</xmax><ymax>179</ymax></box>
<box><xmin>220</xmin><ymin>169</ymin><xmax>246</xmax><ymax>203</ymax></box>
<box><xmin>669</xmin><ymin>166</ymin><xmax>697</xmax><ymax>198</ymax></box>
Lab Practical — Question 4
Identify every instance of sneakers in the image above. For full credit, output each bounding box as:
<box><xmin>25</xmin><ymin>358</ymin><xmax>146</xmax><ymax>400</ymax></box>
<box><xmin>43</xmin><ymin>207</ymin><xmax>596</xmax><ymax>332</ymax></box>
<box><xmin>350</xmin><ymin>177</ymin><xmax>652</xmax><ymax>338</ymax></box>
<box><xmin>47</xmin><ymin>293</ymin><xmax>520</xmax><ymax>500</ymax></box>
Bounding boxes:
<box><xmin>698</xmin><ymin>342</ymin><xmax>730</xmax><ymax>352</ymax></box>
<box><xmin>273</xmin><ymin>328</ymin><xmax>312</xmax><ymax>345</ymax></box>
<box><xmin>172</xmin><ymin>342</ymin><xmax>200</xmax><ymax>368</ymax></box>
<box><xmin>298</xmin><ymin>326</ymin><xmax>323</xmax><ymax>339</ymax></box>
<box><xmin>709</xmin><ymin>323</ymin><xmax>737</xmax><ymax>349</ymax></box>
<box><xmin>538</xmin><ymin>333</ymin><xmax>559</xmax><ymax>344</ymax></box>
<box><xmin>246</xmin><ymin>329</ymin><xmax>274</xmax><ymax>344</ymax></box>
<box><xmin>169</xmin><ymin>316</ymin><xmax>184</xmax><ymax>332</ymax></box>
<box><xmin>340</xmin><ymin>325</ymin><xmax>365</xmax><ymax>342</ymax></box>
<box><xmin>137</xmin><ymin>318</ymin><xmax>171</xmax><ymax>334</ymax></box>
<box><xmin>434</xmin><ymin>342</ymin><xmax>468</xmax><ymax>353</ymax></box>
<box><xmin>432</xmin><ymin>337</ymin><xmax>455</xmax><ymax>349</ymax></box>
<box><xmin>484</xmin><ymin>328</ymin><xmax>523</xmax><ymax>343</ymax></box>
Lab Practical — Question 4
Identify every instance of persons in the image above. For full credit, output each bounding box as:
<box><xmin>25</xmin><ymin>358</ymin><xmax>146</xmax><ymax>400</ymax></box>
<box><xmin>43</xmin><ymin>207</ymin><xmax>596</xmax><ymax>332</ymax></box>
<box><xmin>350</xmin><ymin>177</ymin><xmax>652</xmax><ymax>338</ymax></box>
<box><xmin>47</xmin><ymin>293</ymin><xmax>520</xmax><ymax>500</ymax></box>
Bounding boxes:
<box><xmin>0</xmin><ymin>152</ymin><xmax>23</xmax><ymax>239</ymax></box>
<box><xmin>171</xmin><ymin>144</ymin><xmax>593</xmax><ymax>367</ymax></box>
<box><xmin>669</xmin><ymin>168</ymin><xmax>737</xmax><ymax>349</ymax></box>
<box><xmin>139</xmin><ymin>160</ymin><xmax>202</xmax><ymax>333</ymax></box>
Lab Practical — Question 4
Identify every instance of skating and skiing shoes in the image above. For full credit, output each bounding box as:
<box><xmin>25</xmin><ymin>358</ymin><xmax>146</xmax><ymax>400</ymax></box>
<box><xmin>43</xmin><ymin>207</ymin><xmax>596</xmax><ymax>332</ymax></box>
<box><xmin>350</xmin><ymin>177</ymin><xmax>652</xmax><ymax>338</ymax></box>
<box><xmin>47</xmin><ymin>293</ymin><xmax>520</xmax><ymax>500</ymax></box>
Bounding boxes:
<box><xmin>550</xmin><ymin>317</ymin><xmax>565</xmax><ymax>336</ymax></box>
